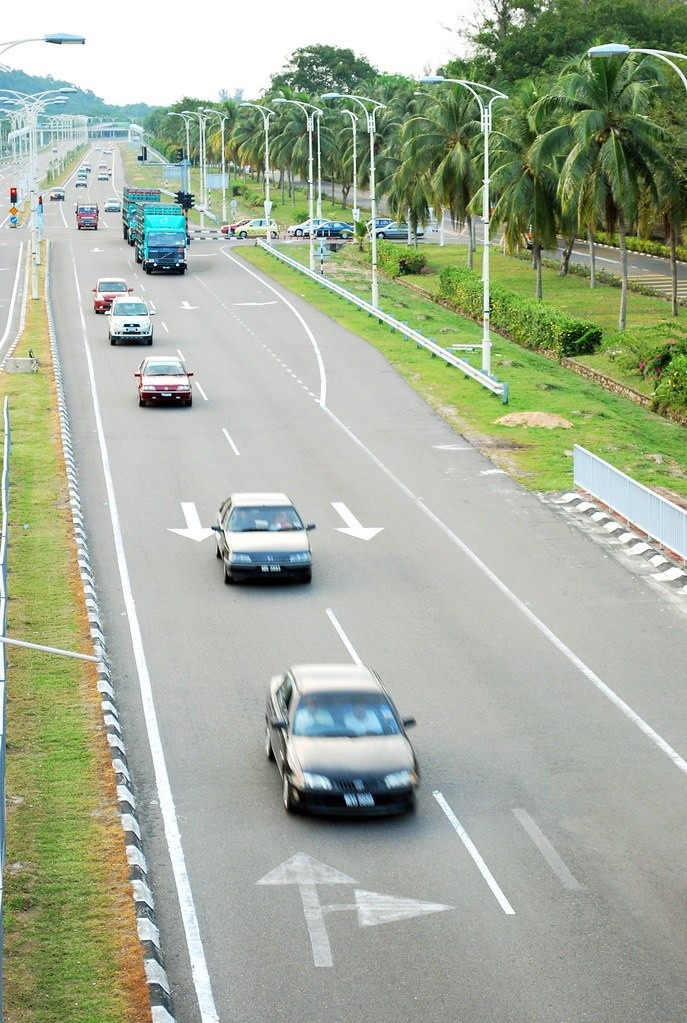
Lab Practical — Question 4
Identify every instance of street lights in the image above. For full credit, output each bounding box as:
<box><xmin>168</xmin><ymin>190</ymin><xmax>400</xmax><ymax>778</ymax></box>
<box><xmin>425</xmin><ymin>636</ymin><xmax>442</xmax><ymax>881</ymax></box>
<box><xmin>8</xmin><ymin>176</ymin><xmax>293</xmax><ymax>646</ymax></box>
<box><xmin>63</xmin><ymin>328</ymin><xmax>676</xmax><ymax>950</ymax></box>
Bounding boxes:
<box><xmin>418</xmin><ymin>76</ymin><xmax>509</xmax><ymax>377</ymax></box>
<box><xmin>1</xmin><ymin>85</ymin><xmax>77</xmax><ymax>187</ymax></box>
<box><xmin>181</xmin><ymin>110</ymin><xmax>212</xmax><ymax>211</ymax></box>
<box><xmin>238</xmin><ymin>102</ymin><xmax>275</xmax><ymax>245</ymax></box>
<box><xmin>270</xmin><ymin>98</ymin><xmax>325</xmax><ymax>269</ymax></box>
<box><xmin>167</xmin><ymin>112</ymin><xmax>195</xmax><ymax>195</ymax></box>
<box><xmin>321</xmin><ymin>92</ymin><xmax>388</xmax><ymax>310</ymax></box>
<box><xmin>197</xmin><ymin>106</ymin><xmax>230</xmax><ymax>226</ymax></box>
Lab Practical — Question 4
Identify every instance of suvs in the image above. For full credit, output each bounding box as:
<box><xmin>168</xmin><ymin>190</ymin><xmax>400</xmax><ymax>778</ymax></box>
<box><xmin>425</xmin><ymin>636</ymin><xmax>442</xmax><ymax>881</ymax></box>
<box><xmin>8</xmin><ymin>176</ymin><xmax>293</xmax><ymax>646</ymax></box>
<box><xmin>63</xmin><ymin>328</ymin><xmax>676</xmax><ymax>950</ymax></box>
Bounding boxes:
<box><xmin>104</xmin><ymin>295</ymin><xmax>155</xmax><ymax>347</ymax></box>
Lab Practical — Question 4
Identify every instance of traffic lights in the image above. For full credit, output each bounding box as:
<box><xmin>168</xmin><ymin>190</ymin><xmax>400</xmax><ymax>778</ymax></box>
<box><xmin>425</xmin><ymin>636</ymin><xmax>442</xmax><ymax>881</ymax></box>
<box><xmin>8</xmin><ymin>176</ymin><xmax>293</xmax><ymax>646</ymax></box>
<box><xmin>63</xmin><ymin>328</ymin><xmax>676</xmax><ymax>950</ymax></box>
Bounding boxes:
<box><xmin>137</xmin><ymin>156</ymin><xmax>143</xmax><ymax>161</ymax></box>
<box><xmin>174</xmin><ymin>192</ymin><xmax>182</xmax><ymax>204</ymax></box>
<box><xmin>10</xmin><ymin>188</ymin><xmax>17</xmax><ymax>203</ymax></box>
<box><xmin>188</xmin><ymin>194</ymin><xmax>195</xmax><ymax>209</ymax></box>
<box><xmin>182</xmin><ymin>195</ymin><xmax>188</xmax><ymax>209</ymax></box>
<box><xmin>176</xmin><ymin>148</ymin><xmax>183</xmax><ymax>162</ymax></box>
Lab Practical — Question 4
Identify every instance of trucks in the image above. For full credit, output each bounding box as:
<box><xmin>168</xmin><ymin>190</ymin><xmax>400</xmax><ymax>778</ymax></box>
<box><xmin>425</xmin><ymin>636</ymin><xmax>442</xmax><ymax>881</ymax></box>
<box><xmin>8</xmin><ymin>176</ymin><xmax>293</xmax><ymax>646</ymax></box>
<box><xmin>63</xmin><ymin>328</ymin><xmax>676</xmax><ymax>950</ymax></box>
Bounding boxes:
<box><xmin>75</xmin><ymin>203</ymin><xmax>99</xmax><ymax>230</ymax></box>
<box><xmin>133</xmin><ymin>201</ymin><xmax>191</xmax><ymax>275</ymax></box>
<box><xmin>123</xmin><ymin>187</ymin><xmax>161</xmax><ymax>247</ymax></box>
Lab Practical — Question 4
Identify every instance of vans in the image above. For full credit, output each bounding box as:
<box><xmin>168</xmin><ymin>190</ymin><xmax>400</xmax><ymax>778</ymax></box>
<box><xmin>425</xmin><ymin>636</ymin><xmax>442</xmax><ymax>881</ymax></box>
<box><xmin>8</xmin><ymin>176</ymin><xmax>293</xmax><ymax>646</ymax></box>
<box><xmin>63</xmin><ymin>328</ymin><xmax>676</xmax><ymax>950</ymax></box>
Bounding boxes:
<box><xmin>367</xmin><ymin>218</ymin><xmax>392</xmax><ymax>232</ymax></box>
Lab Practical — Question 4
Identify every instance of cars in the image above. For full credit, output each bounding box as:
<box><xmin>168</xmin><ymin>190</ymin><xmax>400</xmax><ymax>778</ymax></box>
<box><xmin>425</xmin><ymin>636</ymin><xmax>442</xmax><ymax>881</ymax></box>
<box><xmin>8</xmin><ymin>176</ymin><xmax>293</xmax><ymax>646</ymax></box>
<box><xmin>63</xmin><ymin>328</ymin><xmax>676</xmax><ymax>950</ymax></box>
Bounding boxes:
<box><xmin>81</xmin><ymin>162</ymin><xmax>91</xmax><ymax>172</ymax></box>
<box><xmin>134</xmin><ymin>357</ymin><xmax>195</xmax><ymax>408</ymax></box>
<box><xmin>77</xmin><ymin>169</ymin><xmax>87</xmax><ymax>179</ymax></box>
<box><xmin>370</xmin><ymin>221</ymin><xmax>425</xmax><ymax>239</ymax></box>
<box><xmin>97</xmin><ymin>171</ymin><xmax>108</xmax><ymax>181</ymax></box>
<box><xmin>91</xmin><ymin>144</ymin><xmax>114</xmax><ymax>168</ymax></box>
<box><xmin>302</xmin><ymin>221</ymin><xmax>353</xmax><ymax>239</ymax></box>
<box><xmin>234</xmin><ymin>219</ymin><xmax>279</xmax><ymax>239</ymax></box>
<box><xmin>211</xmin><ymin>492</ymin><xmax>317</xmax><ymax>586</ymax></box>
<box><xmin>49</xmin><ymin>187</ymin><xmax>65</xmax><ymax>201</ymax></box>
<box><xmin>93</xmin><ymin>278</ymin><xmax>134</xmax><ymax>315</ymax></box>
<box><xmin>263</xmin><ymin>661</ymin><xmax>420</xmax><ymax>819</ymax></box>
<box><xmin>75</xmin><ymin>177</ymin><xmax>87</xmax><ymax>187</ymax></box>
<box><xmin>221</xmin><ymin>218</ymin><xmax>253</xmax><ymax>236</ymax></box>
<box><xmin>287</xmin><ymin>218</ymin><xmax>330</xmax><ymax>237</ymax></box>
<box><xmin>104</xmin><ymin>198</ymin><xmax>121</xmax><ymax>212</ymax></box>
<box><xmin>517</xmin><ymin>224</ymin><xmax>545</xmax><ymax>249</ymax></box>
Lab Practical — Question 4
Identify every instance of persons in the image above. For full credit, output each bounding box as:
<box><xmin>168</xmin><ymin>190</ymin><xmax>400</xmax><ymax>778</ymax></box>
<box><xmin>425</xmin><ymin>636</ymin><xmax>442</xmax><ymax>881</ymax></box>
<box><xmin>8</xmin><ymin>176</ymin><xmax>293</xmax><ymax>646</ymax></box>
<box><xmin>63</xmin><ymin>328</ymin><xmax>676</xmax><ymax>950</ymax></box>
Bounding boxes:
<box><xmin>116</xmin><ymin>304</ymin><xmax>146</xmax><ymax>313</ymax></box>
<box><xmin>344</xmin><ymin>697</ymin><xmax>384</xmax><ymax>734</ymax></box>
<box><xmin>294</xmin><ymin>695</ymin><xmax>336</xmax><ymax>734</ymax></box>
<box><xmin>147</xmin><ymin>363</ymin><xmax>182</xmax><ymax>375</ymax></box>
<box><xmin>269</xmin><ymin>510</ymin><xmax>292</xmax><ymax>530</ymax></box>
<box><xmin>100</xmin><ymin>283</ymin><xmax>124</xmax><ymax>292</ymax></box>
<box><xmin>231</xmin><ymin>509</ymin><xmax>256</xmax><ymax>530</ymax></box>
